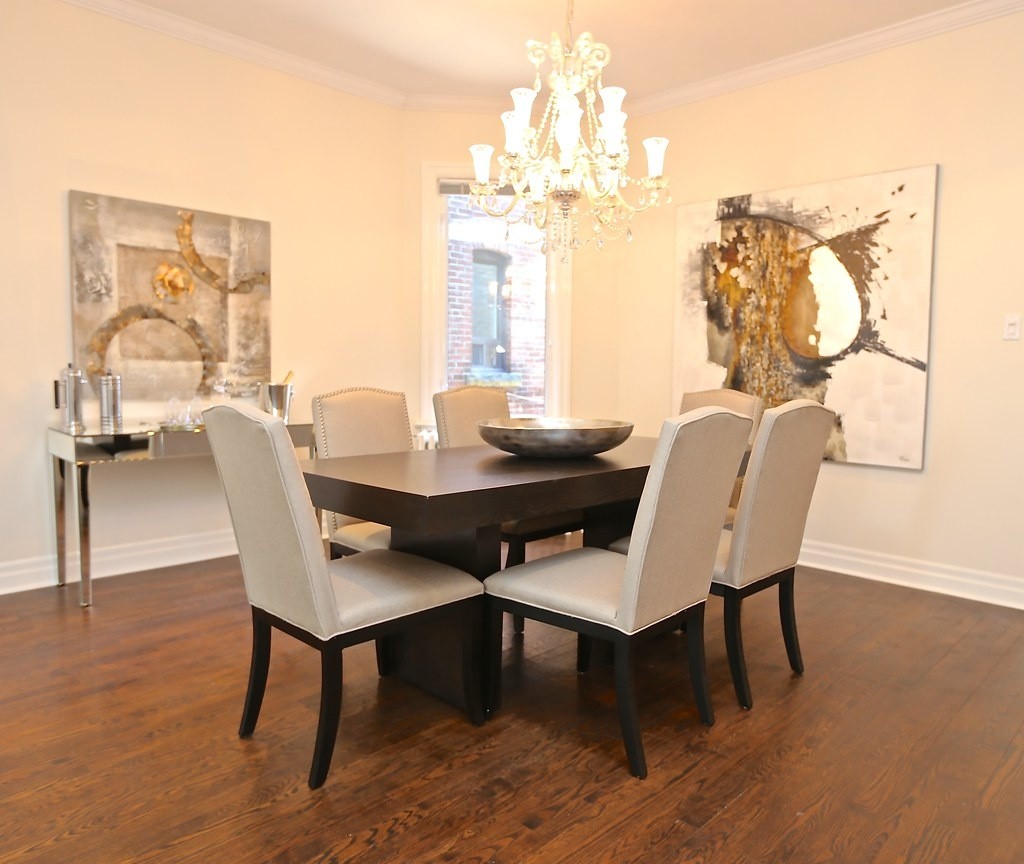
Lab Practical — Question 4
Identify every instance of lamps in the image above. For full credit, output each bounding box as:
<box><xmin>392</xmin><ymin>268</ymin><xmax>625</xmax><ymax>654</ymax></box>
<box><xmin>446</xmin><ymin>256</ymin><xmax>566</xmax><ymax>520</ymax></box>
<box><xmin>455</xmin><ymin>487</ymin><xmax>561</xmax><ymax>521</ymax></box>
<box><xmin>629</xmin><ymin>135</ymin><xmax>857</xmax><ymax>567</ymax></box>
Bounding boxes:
<box><xmin>470</xmin><ymin>0</ymin><xmax>674</xmax><ymax>264</ymax></box>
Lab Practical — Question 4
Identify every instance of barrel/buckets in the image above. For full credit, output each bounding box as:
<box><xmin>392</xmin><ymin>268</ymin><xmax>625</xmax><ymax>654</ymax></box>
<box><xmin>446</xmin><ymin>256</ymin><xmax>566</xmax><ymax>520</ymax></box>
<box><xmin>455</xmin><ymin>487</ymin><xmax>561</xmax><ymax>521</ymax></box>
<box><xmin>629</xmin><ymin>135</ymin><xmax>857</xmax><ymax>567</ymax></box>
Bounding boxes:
<box><xmin>264</xmin><ymin>382</ymin><xmax>294</xmax><ymax>424</ymax></box>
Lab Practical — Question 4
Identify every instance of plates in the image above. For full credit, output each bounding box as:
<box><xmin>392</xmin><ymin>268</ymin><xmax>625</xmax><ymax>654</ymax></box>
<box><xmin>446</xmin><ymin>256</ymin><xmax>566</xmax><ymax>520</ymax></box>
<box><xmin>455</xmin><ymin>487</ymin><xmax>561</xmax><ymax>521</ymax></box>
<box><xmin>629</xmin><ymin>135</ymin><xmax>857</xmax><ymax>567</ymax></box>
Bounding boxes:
<box><xmin>476</xmin><ymin>418</ymin><xmax>634</xmax><ymax>460</ymax></box>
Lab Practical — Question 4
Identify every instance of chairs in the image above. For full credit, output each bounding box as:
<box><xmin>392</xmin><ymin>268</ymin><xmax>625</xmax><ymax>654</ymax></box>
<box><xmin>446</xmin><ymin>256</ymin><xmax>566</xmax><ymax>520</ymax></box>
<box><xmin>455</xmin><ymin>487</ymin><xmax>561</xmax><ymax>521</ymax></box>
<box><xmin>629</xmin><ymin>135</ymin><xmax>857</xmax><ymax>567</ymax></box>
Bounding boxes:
<box><xmin>484</xmin><ymin>406</ymin><xmax>752</xmax><ymax>780</ymax></box>
<box><xmin>609</xmin><ymin>399</ymin><xmax>836</xmax><ymax>709</ymax></box>
<box><xmin>432</xmin><ymin>385</ymin><xmax>525</xmax><ymax>631</ymax></box>
<box><xmin>680</xmin><ymin>389</ymin><xmax>764</xmax><ymax>531</ymax></box>
<box><xmin>201</xmin><ymin>402</ymin><xmax>488</xmax><ymax>788</ymax></box>
<box><xmin>311</xmin><ymin>385</ymin><xmax>414</xmax><ymax>562</ymax></box>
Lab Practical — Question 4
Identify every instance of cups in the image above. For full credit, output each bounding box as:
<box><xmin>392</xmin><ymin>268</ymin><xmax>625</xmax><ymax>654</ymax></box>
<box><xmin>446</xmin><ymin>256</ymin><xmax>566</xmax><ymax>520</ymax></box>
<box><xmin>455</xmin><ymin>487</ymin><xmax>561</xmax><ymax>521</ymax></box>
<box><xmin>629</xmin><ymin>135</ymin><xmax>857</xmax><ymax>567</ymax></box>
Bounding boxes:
<box><xmin>164</xmin><ymin>394</ymin><xmax>191</xmax><ymax>427</ymax></box>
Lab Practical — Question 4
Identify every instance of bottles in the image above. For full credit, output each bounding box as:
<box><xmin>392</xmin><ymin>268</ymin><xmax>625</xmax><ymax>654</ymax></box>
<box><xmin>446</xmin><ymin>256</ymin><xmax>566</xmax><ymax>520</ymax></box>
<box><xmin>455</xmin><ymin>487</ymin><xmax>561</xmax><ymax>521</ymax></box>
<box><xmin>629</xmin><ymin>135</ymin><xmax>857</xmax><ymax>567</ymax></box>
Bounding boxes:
<box><xmin>59</xmin><ymin>363</ymin><xmax>82</xmax><ymax>427</ymax></box>
<box><xmin>100</xmin><ymin>369</ymin><xmax>123</xmax><ymax>426</ymax></box>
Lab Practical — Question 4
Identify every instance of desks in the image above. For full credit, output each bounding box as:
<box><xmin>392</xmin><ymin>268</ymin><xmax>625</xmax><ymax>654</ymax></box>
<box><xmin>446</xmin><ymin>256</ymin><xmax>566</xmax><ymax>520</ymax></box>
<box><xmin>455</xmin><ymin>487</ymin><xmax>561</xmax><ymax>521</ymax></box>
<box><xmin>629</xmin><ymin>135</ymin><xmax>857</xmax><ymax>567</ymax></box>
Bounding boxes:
<box><xmin>298</xmin><ymin>436</ymin><xmax>752</xmax><ymax>725</ymax></box>
<box><xmin>48</xmin><ymin>418</ymin><xmax>317</xmax><ymax>608</ymax></box>
